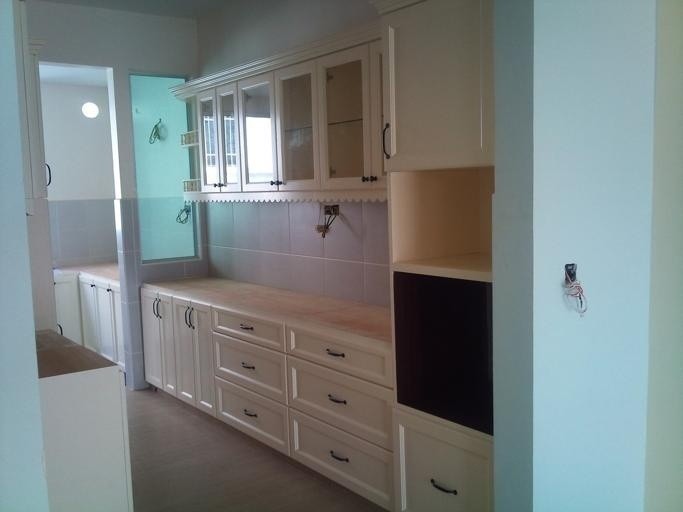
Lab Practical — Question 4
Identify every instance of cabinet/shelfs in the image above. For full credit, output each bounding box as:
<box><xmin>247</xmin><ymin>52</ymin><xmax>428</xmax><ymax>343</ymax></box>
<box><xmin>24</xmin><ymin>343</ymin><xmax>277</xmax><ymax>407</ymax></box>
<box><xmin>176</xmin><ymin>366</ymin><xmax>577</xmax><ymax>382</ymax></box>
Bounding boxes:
<box><xmin>137</xmin><ymin>289</ymin><xmax>215</xmax><ymax>417</ymax></box>
<box><xmin>284</xmin><ymin>328</ymin><xmax>390</xmax><ymax>509</ymax></box>
<box><xmin>55</xmin><ymin>277</ymin><xmax>123</xmax><ymax>367</ymax></box>
<box><xmin>210</xmin><ymin>311</ymin><xmax>289</xmax><ymax>457</ymax></box>
<box><xmin>385</xmin><ymin>4</ymin><xmax>495</xmax><ymax>169</ymax></box>
<box><xmin>169</xmin><ymin>26</ymin><xmax>385</xmax><ymax>204</ymax></box>
<box><xmin>395</xmin><ymin>416</ymin><xmax>492</xmax><ymax>511</ymax></box>
